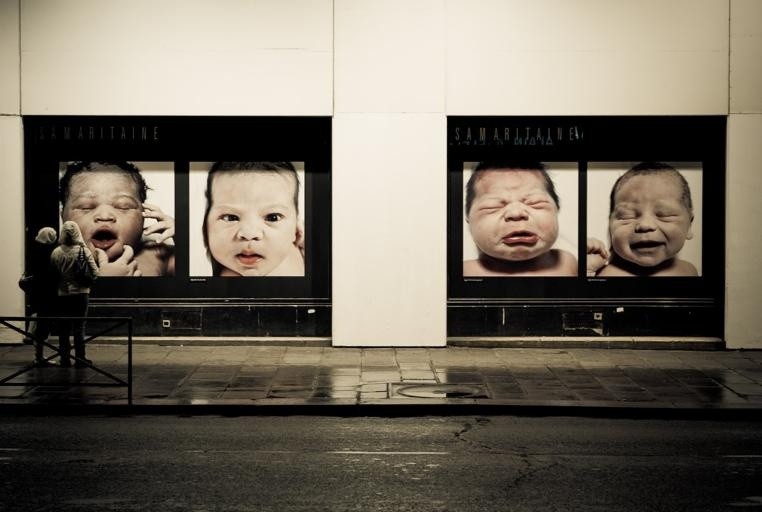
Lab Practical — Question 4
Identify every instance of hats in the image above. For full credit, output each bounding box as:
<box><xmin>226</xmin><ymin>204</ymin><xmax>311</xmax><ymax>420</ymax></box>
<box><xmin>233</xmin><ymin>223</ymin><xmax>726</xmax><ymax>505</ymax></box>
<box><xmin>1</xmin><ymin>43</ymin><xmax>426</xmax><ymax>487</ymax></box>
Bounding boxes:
<box><xmin>35</xmin><ymin>226</ymin><xmax>57</xmax><ymax>244</ymax></box>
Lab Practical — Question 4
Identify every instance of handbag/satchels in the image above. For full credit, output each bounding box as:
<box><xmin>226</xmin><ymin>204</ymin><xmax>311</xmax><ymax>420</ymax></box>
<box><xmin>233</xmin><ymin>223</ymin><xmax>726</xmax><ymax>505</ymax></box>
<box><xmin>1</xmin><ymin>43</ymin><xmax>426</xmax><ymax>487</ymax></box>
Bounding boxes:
<box><xmin>19</xmin><ymin>276</ymin><xmax>34</xmax><ymax>296</ymax></box>
<box><xmin>74</xmin><ymin>259</ymin><xmax>100</xmax><ymax>292</ymax></box>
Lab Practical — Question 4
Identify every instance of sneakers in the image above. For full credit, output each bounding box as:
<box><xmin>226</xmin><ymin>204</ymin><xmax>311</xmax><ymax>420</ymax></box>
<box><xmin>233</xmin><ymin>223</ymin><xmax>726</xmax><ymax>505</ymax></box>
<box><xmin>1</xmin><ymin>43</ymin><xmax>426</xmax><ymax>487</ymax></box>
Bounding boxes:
<box><xmin>34</xmin><ymin>355</ymin><xmax>92</xmax><ymax>369</ymax></box>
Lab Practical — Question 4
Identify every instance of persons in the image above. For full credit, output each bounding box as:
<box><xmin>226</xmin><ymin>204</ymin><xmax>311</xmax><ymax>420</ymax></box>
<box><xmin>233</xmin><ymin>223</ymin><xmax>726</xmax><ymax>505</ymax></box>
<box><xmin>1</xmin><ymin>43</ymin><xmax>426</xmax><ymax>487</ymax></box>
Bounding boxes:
<box><xmin>59</xmin><ymin>160</ymin><xmax>176</xmax><ymax>279</ymax></box>
<box><xmin>200</xmin><ymin>161</ymin><xmax>304</xmax><ymax>278</ymax></box>
<box><xmin>49</xmin><ymin>219</ymin><xmax>99</xmax><ymax>368</ymax></box>
<box><xmin>585</xmin><ymin>160</ymin><xmax>707</xmax><ymax>282</ymax></box>
<box><xmin>26</xmin><ymin>226</ymin><xmax>60</xmax><ymax>368</ymax></box>
<box><xmin>464</xmin><ymin>159</ymin><xmax>580</xmax><ymax>278</ymax></box>
